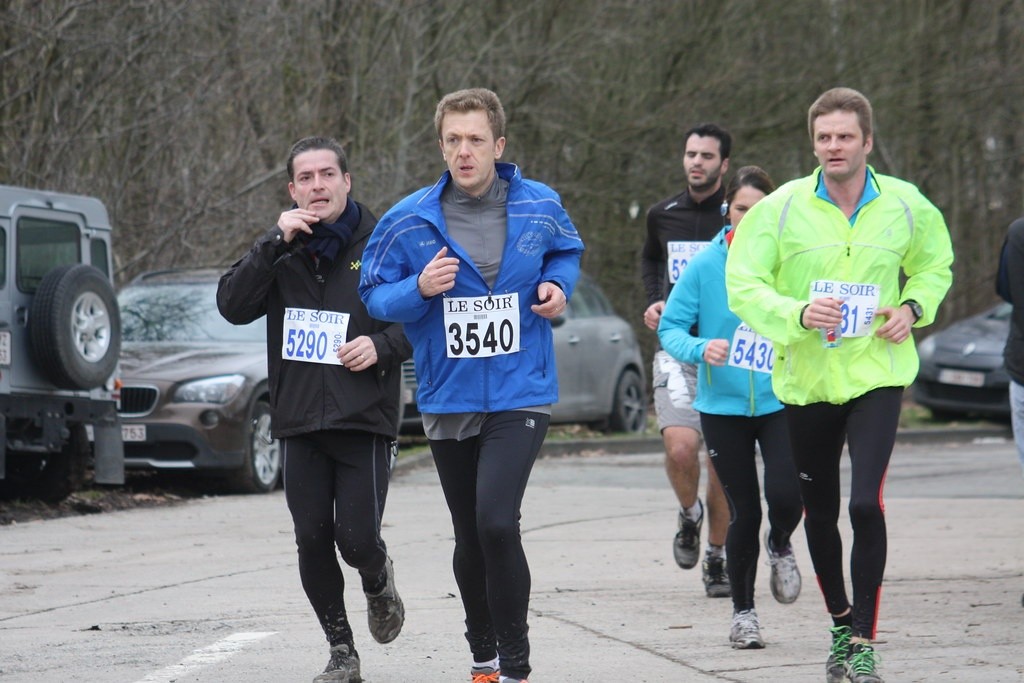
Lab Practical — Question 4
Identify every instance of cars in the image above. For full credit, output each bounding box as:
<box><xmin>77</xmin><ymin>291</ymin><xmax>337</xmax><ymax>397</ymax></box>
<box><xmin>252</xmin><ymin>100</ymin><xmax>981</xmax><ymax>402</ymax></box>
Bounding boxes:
<box><xmin>913</xmin><ymin>295</ymin><xmax>1013</xmax><ymax>426</ymax></box>
<box><xmin>397</xmin><ymin>270</ymin><xmax>650</xmax><ymax>438</ymax></box>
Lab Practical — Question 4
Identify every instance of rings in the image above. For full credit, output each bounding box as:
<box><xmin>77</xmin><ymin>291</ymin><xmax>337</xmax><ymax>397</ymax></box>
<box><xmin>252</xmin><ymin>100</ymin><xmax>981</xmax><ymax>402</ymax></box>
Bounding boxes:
<box><xmin>555</xmin><ymin>308</ymin><xmax>558</xmax><ymax>311</ymax></box>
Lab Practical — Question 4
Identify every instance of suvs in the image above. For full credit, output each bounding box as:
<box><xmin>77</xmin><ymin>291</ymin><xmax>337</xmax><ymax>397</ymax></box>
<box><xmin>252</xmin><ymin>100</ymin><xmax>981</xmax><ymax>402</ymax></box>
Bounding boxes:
<box><xmin>110</xmin><ymin>266</ymin><xmax>283</xmax><ymax>493</ymax></box>
<box><xmin>1</xmin><ymin>184</ymin><xmax>123</xmax><ymax>508</ymax></box>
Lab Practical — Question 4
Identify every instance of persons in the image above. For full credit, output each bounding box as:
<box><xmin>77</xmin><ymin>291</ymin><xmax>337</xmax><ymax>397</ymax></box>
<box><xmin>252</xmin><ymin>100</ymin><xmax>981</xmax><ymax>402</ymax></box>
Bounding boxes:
<box><xmin>725</xmin><ymin>88</ymin><xmax>956</xmax><ymax>683</ymax></box>
<box><xmin>217</xmin><ymin>136</ymin><xmax>412</xmax><ymax>682</ymax></box>
<box><xmin>995</xmin><ymin>212</ymin><xmax>1024</xmax><ymax>610</ymax></box>
<box><xmin>359</xmin><ymin>90</ymin><xmax>622</xmax><ymax>682</ymax></box>
<box><xmin>642</xmin><ymin>125</ymin><xmax>804</xmax><ymax>650</ymax></box>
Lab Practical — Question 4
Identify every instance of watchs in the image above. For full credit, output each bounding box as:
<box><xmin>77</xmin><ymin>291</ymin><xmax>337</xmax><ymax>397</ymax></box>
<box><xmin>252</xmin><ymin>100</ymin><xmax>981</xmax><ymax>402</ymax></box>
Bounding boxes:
<box><xmin>902</xmin><ymin>299</ymin><xmax>923</xmax><ymax>323</ymax></box>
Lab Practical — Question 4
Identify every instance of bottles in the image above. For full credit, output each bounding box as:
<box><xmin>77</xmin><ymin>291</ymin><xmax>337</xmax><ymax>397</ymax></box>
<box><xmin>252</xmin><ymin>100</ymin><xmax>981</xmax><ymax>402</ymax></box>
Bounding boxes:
<box><xmin>821</xmin><ymin>298</ymin><xmax>842</xmax><ymax>348</ymax></box>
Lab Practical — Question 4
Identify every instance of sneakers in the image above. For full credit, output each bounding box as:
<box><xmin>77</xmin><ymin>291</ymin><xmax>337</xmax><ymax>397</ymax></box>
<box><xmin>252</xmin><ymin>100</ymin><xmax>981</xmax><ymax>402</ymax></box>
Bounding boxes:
<box><xmin>312</xmin><ymin>644</ymin><xmax>361</xmax><ymax>683</ymax></box>
<box><xmin>673</xmin><ymin>497</ymin><xmax>704</xmax><ymax>569</ymax></box>
<box><xmin>470</xmin><ymin>666</ymin><xmax>500</xmax><ymax>683</ymax></box>
<box><xmin>762</xmin><ymin>529</ymin><xmax>801</xmax><ymax>603</ymax></box>
<box><xmin>366</xmin><ymin>555</ymin><xmax>405</xmax><ymax>644</ymax></box>
<box><xmin>843</xmin><ymin>644</ymin><xmax>884</xmax><ymax>683</ymax></box>
<box><xmin>702</xmin><ymin>556</ymin><xmax>731</xmax><ymax>595</ymax></box>
<box><xmin>730</xmin><ymin>608</ymin><xmax>764</xmax><ymax>649</ymax></box>
<box><xmin>827</xmin><ymin>625</ymin><xmax>852</xmax><ymax>683</ymax></box>
